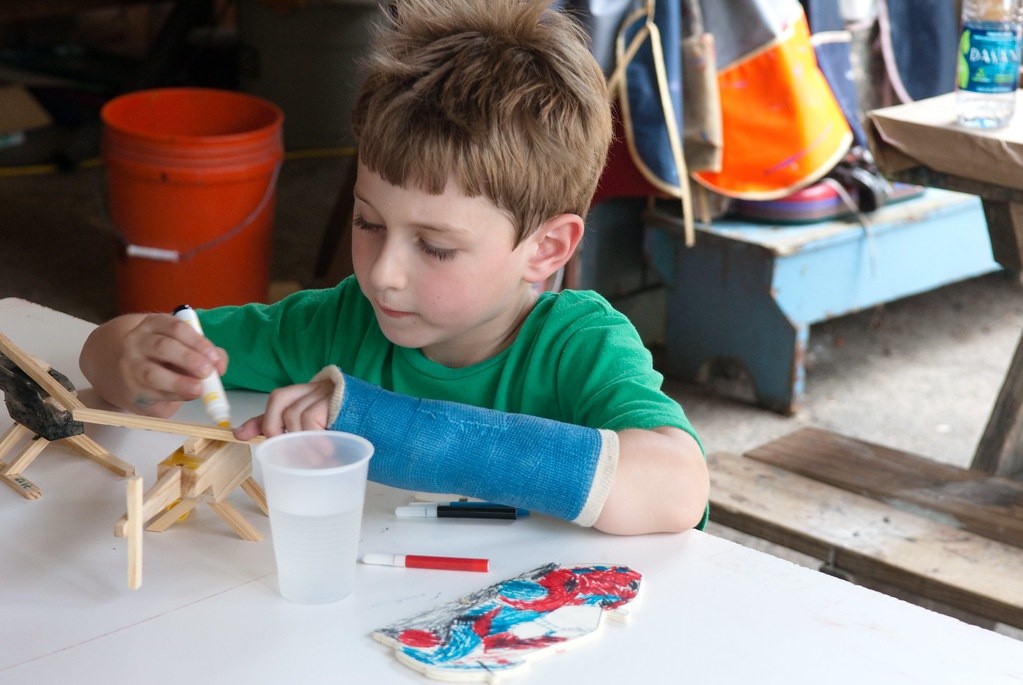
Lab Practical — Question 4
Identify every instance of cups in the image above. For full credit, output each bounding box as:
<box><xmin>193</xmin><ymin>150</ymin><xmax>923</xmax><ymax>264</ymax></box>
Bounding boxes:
<box><xmin>256</xmin><ymin>431</ymin><xmax>376</xmax><ymax>605</ymax></box>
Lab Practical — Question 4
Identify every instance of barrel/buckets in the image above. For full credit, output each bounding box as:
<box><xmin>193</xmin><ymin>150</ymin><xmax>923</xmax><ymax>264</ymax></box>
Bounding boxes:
<box><xmin>98</xmin><ymin>86</ymin><xmax>286</xmax><ymax>323</ymax></box>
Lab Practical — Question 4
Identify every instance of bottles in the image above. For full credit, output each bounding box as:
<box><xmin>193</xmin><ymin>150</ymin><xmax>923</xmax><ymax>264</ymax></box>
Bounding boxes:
<box><xmin>954</xmin><ymin>0</ymin><xmax>1022</xmax><ymax>130</ymax></box>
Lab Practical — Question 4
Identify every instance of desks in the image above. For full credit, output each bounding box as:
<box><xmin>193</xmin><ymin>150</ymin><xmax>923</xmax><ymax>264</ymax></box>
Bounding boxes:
<box><xmin>634</xmin><ymin>173</ymin><xmax>1003</xmax><ymax>419</ymax></box>
<box><xmin>2</xmin><ymin>295</ymin><xmax>1022</xmax><ymax>682</ymax></box>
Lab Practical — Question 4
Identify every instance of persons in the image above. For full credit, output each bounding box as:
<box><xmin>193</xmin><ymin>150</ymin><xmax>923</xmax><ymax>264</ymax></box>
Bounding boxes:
<box><xmin>78</xmin><ymin>1</ymin><xmax>711</xmax><ymax>537</ymax></box>
